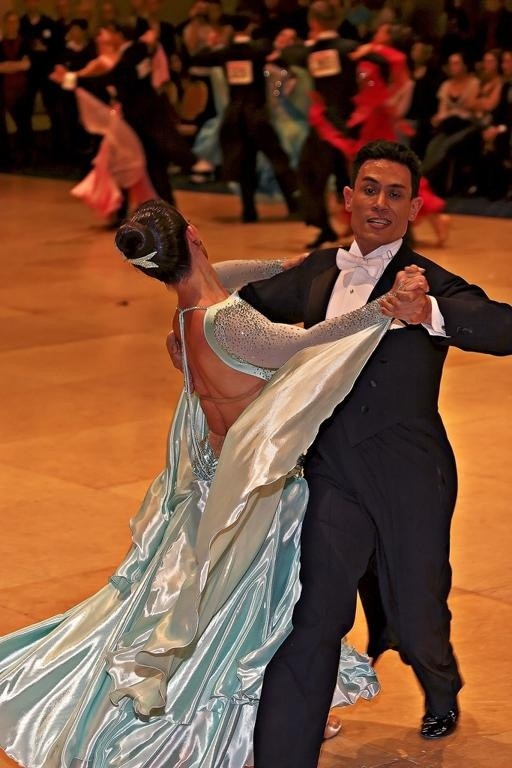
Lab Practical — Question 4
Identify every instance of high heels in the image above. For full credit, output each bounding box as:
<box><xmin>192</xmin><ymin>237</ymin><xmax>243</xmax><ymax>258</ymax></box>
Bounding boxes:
<box><xmin>324</xmin><ymin>714</ymin><xmax>342</xmax><ymax>738</ymax></box>
<box><xmin>436</xmin><ymin>214</ymin><xmax>457</xmax><ymax>248</ymax></box>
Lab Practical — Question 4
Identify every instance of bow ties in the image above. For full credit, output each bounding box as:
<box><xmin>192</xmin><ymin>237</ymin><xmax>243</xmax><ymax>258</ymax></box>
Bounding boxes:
<box><xmin>335</xmin><ymin>247</ymin><xmax>384</xmax><ymax>279</ymax></box>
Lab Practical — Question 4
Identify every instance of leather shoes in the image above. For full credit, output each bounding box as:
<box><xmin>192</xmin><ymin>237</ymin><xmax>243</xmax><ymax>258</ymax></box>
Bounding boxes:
<box><xmin>306</xmin><ymin>233</ymin><xmax>338</xmax><ymax>247</ymax></box>
<box><xmin>420</xmin><ymin>703</ymin><xmax>459</xmax><ymax>739</ymax></box>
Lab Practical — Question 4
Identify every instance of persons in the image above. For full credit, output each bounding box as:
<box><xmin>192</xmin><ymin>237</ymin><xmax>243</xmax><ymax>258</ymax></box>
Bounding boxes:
<box><xmin>204</xmin><ymin>18</ymin><xmax>307</xmax><ymax>220</ymax></box>
<box><xmin>0</xmin><ymin>198</ymin><xmax>430</xmax><ymax>768</ymax></box>
<box><xmin>162</xmin><ymin>138</ymin><xmax>512</xmax><ymax>768</ymax></box>
<box><xmin>53</xmin><ymin>22</ymin><xmax>180</xmax><ymax>231</ymax></box>
<box><xmin>0</xmin><ymin>0</ymin><xmax>512</xmax><ymax>181</ymax></box>
<box><xmin>290</xmin><ymin>2</ymin><xmax>452</xmax><ymax>250</ymax></box>
